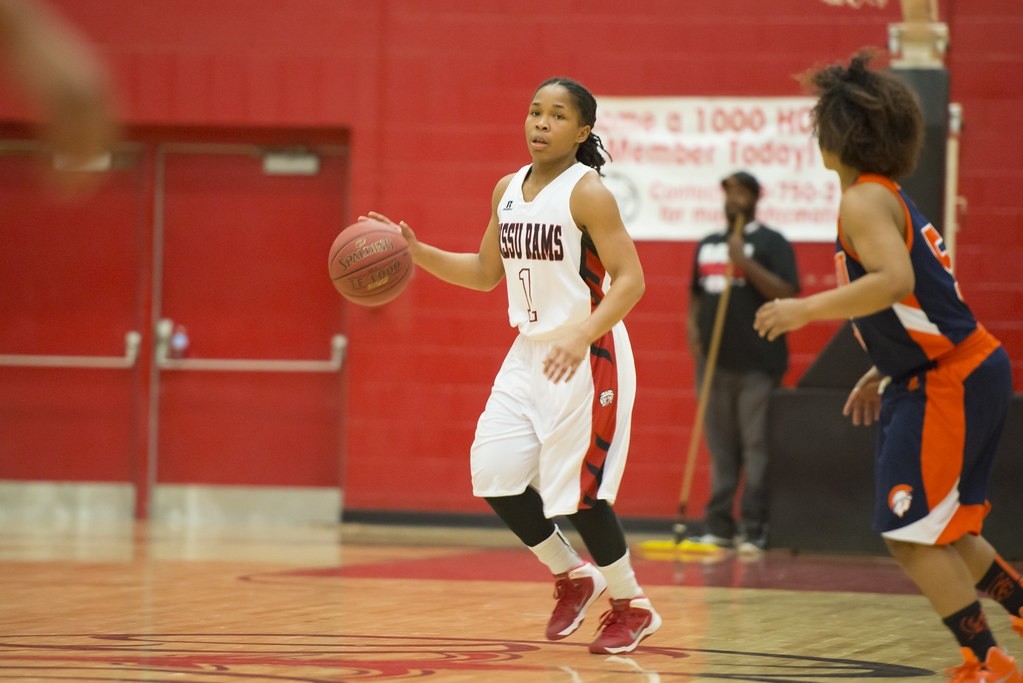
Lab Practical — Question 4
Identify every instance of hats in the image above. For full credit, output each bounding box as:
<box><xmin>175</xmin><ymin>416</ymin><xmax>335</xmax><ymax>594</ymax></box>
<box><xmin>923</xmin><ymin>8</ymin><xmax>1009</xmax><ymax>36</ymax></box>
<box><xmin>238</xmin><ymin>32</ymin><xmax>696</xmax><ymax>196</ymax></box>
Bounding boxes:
<box><xmin>721</xmin><ymin>171</ymin><xmax>760</xmax><ymax>198</ymax></box>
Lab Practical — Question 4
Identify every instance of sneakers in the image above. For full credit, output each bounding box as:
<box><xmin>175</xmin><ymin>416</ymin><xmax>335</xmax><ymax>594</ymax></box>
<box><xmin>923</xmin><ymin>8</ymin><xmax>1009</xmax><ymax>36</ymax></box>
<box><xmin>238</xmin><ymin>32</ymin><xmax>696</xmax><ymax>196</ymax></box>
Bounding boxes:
<box><xmin>1010</xmin><ymin>609</ymin><xmax>1023</xmax><ymax>640</ymax></box>
<box><xmin>546</xmin><ymin>562</ymin><xmax>607</xmax><ymax>640</ymax></box>
<box><xmin>949</xmin><ymin>647</ymin><xmax>1022</xmax><ymax>683</ymax></box>
<box><xmin>590</xmin><ymin>591</ymin><xmax>662</xmax><ymax>655</ymax></box>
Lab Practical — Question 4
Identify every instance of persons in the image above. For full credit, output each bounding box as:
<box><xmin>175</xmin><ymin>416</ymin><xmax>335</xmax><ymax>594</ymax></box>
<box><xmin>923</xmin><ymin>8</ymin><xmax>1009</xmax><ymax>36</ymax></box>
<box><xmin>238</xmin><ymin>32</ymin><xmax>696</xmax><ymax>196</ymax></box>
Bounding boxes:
<box><xmin>0</xmin><ymin>0</ymin><xmax>116</xmax><ymax>205</ymax></box>
<box><xmin>753</xmin><ymin>52</ymin><xmax>1023</xmax><ymax>683</ymax></box>
<box><xmin>688</xmin><ymin>174</ymin><xmax>799</xmax><ymax>554</ymax></box>
<box><xmin>358</xmin><ymin>77</ymin><xmax>660</xmax><ymax>657</ymax></box>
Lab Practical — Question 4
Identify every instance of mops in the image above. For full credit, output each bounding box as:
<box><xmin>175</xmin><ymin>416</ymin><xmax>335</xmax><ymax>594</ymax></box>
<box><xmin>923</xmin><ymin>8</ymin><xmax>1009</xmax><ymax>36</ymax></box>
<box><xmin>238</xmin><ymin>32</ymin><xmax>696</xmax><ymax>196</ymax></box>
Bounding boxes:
<box><xmin>637</xmin><ymin>214</ymin><xmax>744</xmax><ymax>556</ymax></box>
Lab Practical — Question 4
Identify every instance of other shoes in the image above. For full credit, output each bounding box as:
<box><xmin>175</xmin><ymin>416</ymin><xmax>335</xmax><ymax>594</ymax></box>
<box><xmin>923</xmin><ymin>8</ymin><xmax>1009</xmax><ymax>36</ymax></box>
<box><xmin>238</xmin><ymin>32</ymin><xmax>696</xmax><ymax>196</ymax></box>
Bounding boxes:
<box><xmin>693</xmin><ymin>532</ymin><xmax>733</xmax><ymax>547</ymax></box>
<box><xmin>739</xmin><ymin>538</ymin><xmax>761</xmax><ymax>554</ymax></box>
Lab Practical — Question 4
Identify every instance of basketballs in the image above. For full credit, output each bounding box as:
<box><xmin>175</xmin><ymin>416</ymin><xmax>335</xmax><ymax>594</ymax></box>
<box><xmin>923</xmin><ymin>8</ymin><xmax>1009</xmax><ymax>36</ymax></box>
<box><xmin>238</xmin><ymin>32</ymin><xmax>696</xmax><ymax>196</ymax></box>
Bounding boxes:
<box><xmin>327</xmin><ymin>222</ymin><xmax>413</xmax><ymax>308</ymax></box>
